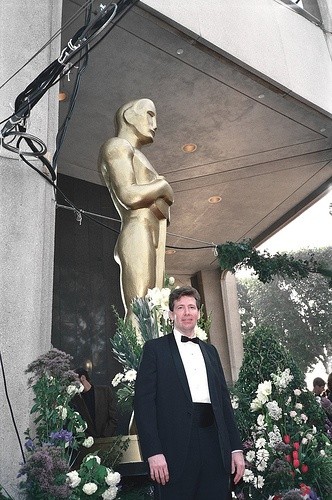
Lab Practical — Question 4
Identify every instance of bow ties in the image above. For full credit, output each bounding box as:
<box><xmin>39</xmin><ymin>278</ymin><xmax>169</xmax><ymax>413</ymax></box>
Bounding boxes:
<box><xmin>315</xmin><ymin>394</ymin><xmax>320</xmax><ymax>396</ymax></box>
<box><xmin>181</xmin><ymin>336</ymin><xmax>199</xmax><ymax>344</ymax></box>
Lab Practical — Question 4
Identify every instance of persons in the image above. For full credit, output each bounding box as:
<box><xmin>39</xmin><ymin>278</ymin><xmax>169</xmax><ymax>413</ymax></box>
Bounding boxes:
<box><xmin>322</xmin><ymin>372</ymin><xmax>332</xmax><ymax>403</ymax></box>
<box><xmin>133</xmin><ymin>285</ymin><xmax>245</xmax><ymax>500</ymax></box>
<box><xmin>312</xmin><ymin>376</ymin><xmax>326</xmax><ymax>408</ymax></box>
<box><xmin>68</xmin><ymin>368</ymin><xmax>120</xmax><ymax>439</ymax></box>
<box><xmin>96</xmin><ymin>97</ymin><xmax>175</xmax><ymax>438</ymax></box>
